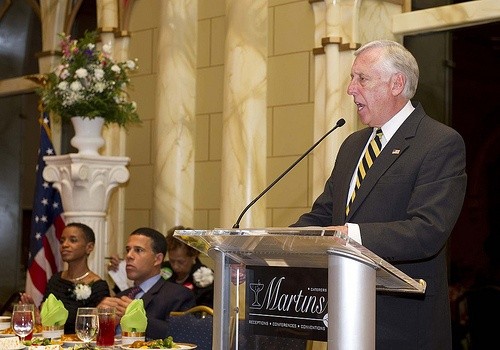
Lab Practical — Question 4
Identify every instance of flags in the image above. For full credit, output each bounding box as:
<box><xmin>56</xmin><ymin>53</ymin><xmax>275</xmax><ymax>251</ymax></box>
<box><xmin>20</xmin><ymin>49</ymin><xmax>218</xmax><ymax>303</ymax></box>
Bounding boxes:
<box><xmin>25</xmin><ymin>102</ymin><xmax>66</xmax><ymax>308</ymax></box>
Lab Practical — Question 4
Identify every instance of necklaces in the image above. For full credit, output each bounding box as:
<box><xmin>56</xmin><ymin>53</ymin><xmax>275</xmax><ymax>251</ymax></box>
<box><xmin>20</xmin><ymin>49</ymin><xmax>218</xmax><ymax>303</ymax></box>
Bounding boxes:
<box><xmin>62</xmin><ymin>270</ymin><xmax>90</xmax><ymax>281</ymax></box>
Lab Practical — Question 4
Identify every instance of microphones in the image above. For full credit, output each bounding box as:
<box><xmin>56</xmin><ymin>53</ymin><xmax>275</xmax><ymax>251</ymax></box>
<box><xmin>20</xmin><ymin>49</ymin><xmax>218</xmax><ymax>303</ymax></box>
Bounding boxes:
<box><xmin>233</xmin><ymin>119</ymin><xmax>345</xmax><ymax>228</ymax></box>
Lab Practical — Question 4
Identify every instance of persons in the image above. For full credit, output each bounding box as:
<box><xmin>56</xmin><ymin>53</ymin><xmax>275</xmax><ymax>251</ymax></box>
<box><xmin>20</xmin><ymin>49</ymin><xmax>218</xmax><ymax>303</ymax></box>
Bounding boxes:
<box><xmin>107</xmin><ymin>225</ymin><xmax>214</xmax><ymax>309</ymax></box>
<box><xmin>93</xmin><ymin>227</ymin><xmax>198</xmax><ymax>339</ymax></box>
<box><xmin>17</xmin><ymin>223</ymin><xmax>110</xmax><ymax>334</ymax></box>
<box><xmin>289</xmin><ymin>41</ymin><xmax>468</xmax><ymax>350</ymax></box>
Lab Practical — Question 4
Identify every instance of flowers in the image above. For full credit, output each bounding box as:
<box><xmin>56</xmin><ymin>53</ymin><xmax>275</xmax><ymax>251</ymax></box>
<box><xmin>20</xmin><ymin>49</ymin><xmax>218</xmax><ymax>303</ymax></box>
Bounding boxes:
<box><xmin>74</xmin><ymin>284</ymin><xmax>92</xmax><ymax>300</ymax></box>
<box><xmin>193</xmin><ymin>266</ymin><xmax>214</xmax><ymax>287</ymax></box>
<box><xmin>25</xmin><ymin>30</ymin><xmax>144</xmax><ymax>131</ymax></box>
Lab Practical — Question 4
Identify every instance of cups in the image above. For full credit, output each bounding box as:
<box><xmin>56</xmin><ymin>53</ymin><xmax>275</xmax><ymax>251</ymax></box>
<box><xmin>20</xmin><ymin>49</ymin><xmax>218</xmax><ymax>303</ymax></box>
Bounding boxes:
<box><xmin>75</xmin><ymin>307</ymin><xmax>99</xmax><ymax>347</ymax></box>
<box><xmin>10</xmin><ymin>304</ymin><xmax>35</xmax><ymax>341</ymax></box>
<box><xmin>96</xmin><ymin>307</ymin><xmax>115</xmax><ymax>350</ymax></box>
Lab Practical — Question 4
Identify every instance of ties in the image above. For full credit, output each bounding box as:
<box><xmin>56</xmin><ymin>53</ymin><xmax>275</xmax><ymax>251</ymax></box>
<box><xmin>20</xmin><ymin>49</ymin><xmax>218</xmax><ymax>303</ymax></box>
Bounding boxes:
<box><xmin>347</xmin><ymin>127</ymin><xmax>385</xmax><ymax>219</ymax></box>
<box><xmin>127</xmin><ymin>286</ymin><xmax>141</xmax><ymax>300</ymax></box>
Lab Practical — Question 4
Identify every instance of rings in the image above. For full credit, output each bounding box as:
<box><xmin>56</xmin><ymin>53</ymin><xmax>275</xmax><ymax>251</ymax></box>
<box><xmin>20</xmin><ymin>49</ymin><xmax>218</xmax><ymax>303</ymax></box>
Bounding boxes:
<box><xmin>107</xmin><ymin>263</ymin><xmax>112</xmax><ymax>267</ymax></box>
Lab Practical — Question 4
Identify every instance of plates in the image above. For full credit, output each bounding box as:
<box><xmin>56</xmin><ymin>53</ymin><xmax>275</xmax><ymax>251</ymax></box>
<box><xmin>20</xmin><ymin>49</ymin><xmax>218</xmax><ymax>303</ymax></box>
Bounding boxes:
<box><xmin>62</xmin><ymin>340</ymin><xmax>122</xmax><ymax>347</ymax></box>
<box><xmin>0</xmin><ymin>333</ymin><xmax>42</xmax><ymax>337</ymax></box>
<box><xmin>7</xmin><ymin>344</ymin><xmax>27</xmax><ymax>350</ymax></box>
<box><xmin>120</xmin><ymin>343</ymin><xmax>198</xmax><ymax>350</ymax></box>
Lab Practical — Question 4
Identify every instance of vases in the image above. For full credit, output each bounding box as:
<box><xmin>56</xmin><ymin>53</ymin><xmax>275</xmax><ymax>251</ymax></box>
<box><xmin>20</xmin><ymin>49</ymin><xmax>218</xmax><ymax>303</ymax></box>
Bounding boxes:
<box><xmin>70</xmin><ymin>115</ymin><xmax>105</xmax><ymax>157</ymax></box>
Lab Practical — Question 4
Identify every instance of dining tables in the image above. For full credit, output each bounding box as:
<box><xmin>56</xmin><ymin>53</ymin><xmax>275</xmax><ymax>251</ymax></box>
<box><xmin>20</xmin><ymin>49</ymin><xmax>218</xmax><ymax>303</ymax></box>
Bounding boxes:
<box><xmin>0</xmin><ymin>317</ymin><xmax>197</xmax><ymax>350</ymax></box>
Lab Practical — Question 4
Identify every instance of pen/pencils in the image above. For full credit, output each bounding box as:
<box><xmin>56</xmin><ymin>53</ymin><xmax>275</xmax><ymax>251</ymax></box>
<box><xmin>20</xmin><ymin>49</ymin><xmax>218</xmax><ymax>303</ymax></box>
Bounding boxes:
<box><xmin>104</xmin><ymin>257</ymin><xmax>124</xmax><ymax>260</ymax></box>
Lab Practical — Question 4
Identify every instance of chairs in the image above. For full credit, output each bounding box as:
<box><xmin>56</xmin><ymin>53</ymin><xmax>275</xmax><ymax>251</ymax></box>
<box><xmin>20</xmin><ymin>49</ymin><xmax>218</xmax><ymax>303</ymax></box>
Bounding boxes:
<box><xmin>168</xmin><ymin>306</ymin><xmax>213</xmax><ymax>350</ymax></box>
